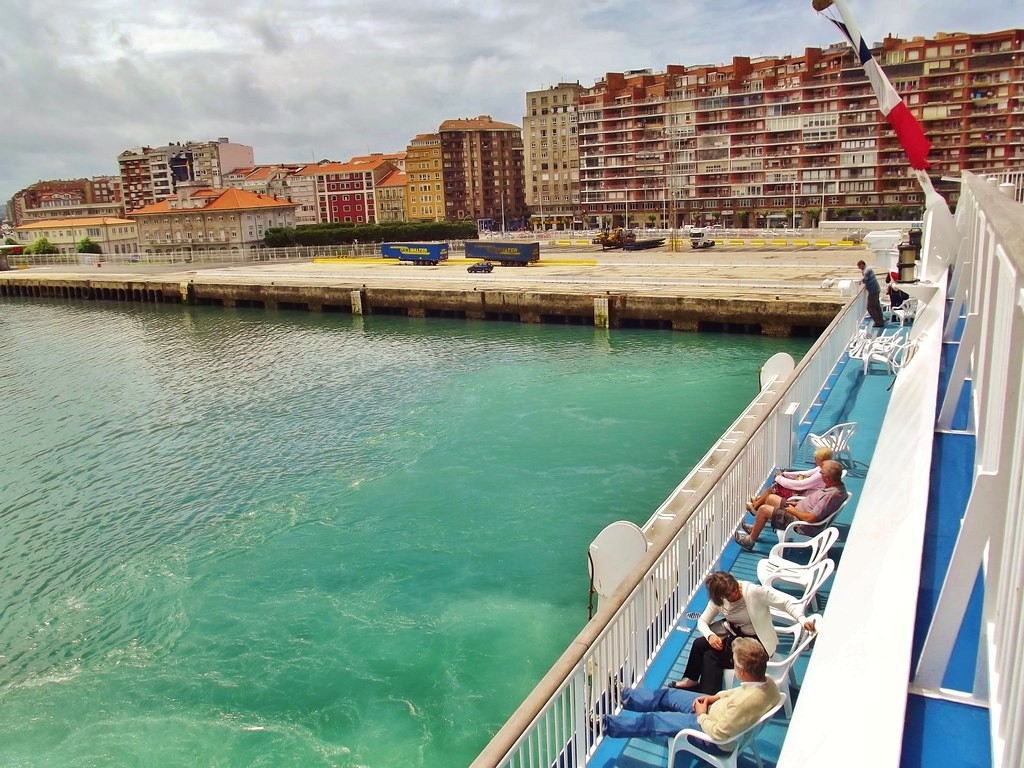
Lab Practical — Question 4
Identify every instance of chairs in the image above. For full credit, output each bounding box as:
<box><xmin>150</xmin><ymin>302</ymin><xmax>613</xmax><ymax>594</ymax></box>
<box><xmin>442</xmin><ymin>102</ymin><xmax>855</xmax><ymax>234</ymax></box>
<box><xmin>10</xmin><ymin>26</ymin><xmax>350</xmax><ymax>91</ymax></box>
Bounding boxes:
<box><xmin>666</xmin><ymin>470</ymin><xmax>855</xmax><ymax>768</ymax></box>
<box><xmin>803</xmin><ymin>422</ymin><xmax>859</xmax><ymax>468</ymax></box>
<box><xmin>844</xmin><ymin>294</ymin><xmax>922</xmax><ymax>376</ymax></box>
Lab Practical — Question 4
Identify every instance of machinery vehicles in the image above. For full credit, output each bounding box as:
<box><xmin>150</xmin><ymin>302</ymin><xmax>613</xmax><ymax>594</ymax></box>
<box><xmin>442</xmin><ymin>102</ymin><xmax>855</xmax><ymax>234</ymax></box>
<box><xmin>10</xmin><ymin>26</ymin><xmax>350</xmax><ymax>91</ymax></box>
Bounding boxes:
<box><xmin>592</xmin><ymin>225</ymin><xmax>636</xmax><ymax>244</ymax></box>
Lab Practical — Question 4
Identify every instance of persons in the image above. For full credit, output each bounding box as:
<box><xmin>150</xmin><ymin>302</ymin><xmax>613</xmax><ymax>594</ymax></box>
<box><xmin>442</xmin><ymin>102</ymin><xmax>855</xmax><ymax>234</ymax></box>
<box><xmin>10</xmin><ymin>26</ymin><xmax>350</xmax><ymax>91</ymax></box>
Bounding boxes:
<box><xmin>733</xmin><ymin>460</ymin><xmax>849</xmax><ymax>552</ymax></box>
<box><xmin>668</xmin><ymin>570</ymin><xmax>815</xmax><ymax>695</ymax></box>
<box><xmin>857</xmin><ymin>260</ymin><xmax>884</xmax><ymax>328</ymax></box>
<box><xmin>746</xmin><ymin>448</ymin><xmax>833</xmax><ymax>516</ymax></box>
<box><xmin>885</xmin><ymin>275</ymin><xmax>902</xmax><ymax>322</ymax></box>
<box><xmin>587</xmin><ymin>637</ymin><xmax>780</xmax><ymax>756</ymax></box>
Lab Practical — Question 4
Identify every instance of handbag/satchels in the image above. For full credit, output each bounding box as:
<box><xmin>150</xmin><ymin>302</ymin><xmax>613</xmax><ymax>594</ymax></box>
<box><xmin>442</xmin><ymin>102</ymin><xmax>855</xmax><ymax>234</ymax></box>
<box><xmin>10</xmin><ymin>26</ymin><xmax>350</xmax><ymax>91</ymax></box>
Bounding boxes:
<box><xmin>795</xmin><ymin>474</ymin><xmax>811</xmax><ymax>493</ymax></box>
<box><xmin>708</xmin><ymin>618</ymin><xmax>742</xmax><ymax>655</ymax></box>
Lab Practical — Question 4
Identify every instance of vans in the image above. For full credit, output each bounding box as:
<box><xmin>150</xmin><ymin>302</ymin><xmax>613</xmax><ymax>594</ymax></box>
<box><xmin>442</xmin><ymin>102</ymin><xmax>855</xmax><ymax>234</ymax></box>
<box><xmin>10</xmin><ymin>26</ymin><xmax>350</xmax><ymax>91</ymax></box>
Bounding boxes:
<box><xmin>690</xmin><ymin>228</ymin><xmax>708</xmax><ymax>242</ymax></box>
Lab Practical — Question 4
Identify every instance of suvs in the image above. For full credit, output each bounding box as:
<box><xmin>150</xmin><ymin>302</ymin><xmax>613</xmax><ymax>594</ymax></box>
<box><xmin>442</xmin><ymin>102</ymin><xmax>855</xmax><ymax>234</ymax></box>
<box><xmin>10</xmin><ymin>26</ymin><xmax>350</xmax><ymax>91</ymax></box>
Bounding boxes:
<box><xmin>467</xmin><ymin>262</ymin><xmax>493</xmax><ymax>274</ymax></box>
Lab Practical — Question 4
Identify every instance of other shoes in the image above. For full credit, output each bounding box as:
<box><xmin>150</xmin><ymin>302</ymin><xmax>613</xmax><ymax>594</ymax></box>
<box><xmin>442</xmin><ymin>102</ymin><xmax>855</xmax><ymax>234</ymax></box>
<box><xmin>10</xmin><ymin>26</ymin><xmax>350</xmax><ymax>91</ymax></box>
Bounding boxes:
<box><xmin>742</xmin><ymin>523</ymin><xmax>753</xmax><ymax>535</ymax></box>
<box><xmin>736</xmin><ymin>529</ymin><xmax>755</xmax><ymax>552</ymax></box>
<box><xmin>668</xmin><ymin>681</ymin><xmax>700</xmax><ymax>690</ymax></box>
<box><xmin>585</xmin><ymin>702</ymin><xmax>603</xmax><ymax>733</ymax></box>
<box><xmin>608</xmin><ymin>670</ymin><xmax>625</xmax><ymax>704</ymax></box>
<box><xmin>747</xmin><ymin>503</ymin><xmax>757</xmax><ymax>516</ymax></box>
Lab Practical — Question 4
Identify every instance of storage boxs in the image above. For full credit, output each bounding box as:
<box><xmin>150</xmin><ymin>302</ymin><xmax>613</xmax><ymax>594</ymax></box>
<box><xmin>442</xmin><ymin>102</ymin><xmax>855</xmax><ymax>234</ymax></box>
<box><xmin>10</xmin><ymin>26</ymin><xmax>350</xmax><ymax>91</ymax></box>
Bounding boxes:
<box><xmin>380</xmin><ymin>241</ymin><xmax>449</xmax><ymax>261</ymax></box>
<box><xmin>464</xmin><ymin>240</ymin><xmax>539</xmax><ymax>261</ymax></box>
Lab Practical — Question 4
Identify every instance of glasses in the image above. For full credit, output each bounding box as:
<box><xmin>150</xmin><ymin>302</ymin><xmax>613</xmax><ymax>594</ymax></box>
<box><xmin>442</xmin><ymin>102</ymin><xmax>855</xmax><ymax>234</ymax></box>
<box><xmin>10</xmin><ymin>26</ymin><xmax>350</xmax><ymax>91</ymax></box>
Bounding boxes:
<box><xmin>820</xmin><ymin>471</ymin><xmax>824</xmax><ymax>474</ymax></box>
<box><xmin>723</xmin><ymin>583</ymin><xmax>737</xmax><ymax>598</ymax></box>
